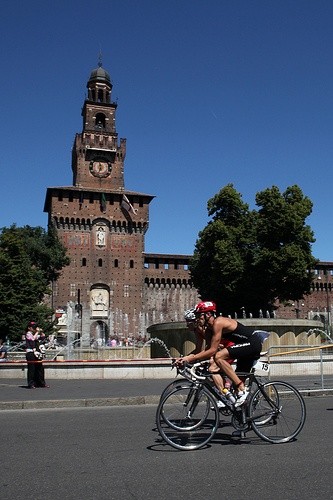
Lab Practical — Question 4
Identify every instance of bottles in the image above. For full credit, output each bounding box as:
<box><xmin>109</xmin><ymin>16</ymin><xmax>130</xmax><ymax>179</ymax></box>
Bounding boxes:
<box><xmin>222</xmin><ymin>388</ymin><xmax>236</xmax><ymax>404</ymax></box>
<box><xmin>224</xmin><ymin>377</ymin><xmax>232</xmax><ymax>392</ymax></box>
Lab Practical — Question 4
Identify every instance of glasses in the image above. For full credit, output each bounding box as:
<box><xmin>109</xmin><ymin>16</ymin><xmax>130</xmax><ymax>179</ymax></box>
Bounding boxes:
<box><xmin>195</xmin><ymin>312</ymin><xmax>208</xmax><ymax>318</ymax></box>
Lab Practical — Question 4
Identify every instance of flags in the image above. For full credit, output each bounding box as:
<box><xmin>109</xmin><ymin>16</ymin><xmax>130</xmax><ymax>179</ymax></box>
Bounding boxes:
<box><xmin>102</xmin><ymin>194</ymin><xmax>106</xmax><ymax>214</ymax></box>
<box><xmin>121</xmin><ymin>194</ymin><xmax>138</xmax><ymax>215</ymax></box>
<box><xmin>79</xmin><ymin>193</ymin><xmax>82</xmax><ymax>210</ymax></box>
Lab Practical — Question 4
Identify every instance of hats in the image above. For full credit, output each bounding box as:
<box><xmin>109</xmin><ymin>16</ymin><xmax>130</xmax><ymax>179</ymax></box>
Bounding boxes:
<box><xmin>28</xmin><ymin>321</ymin><xmax>36</xmax><ymax>327</ymax></box>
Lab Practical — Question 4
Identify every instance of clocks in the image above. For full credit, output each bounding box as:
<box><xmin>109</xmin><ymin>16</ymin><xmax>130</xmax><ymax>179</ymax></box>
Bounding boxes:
<box><xmin>89</xmin><ymin>156</ymin><xmax>112</xmax><ymax>179</ymax></box>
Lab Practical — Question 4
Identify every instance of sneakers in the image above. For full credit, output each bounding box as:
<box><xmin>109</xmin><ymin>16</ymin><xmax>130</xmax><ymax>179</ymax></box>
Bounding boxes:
<box><xmin>211</xmin><ymin>401</ymin><xmax>226</xmax><ymax>411</ymax></box>
<box><xmin>235</xmin><ymin>387</ymin><xmax>249</xmax><ymax>408</ymax></box>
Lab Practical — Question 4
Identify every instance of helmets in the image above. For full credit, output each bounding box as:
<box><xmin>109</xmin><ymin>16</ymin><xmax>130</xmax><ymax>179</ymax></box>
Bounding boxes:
<box><xmin>193</xmin><ymin>301</ymin><xmax>216</xmax><ymax>314</ymax></box>
<box><xmin>184</xmin><ymin>306</ymin><xmax>197</xmax><ymax>319</ymax></box>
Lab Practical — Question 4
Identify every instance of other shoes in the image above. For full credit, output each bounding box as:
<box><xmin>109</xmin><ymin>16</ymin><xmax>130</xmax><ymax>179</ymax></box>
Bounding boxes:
<box><xmin>30</xmin><ymin>385</ymin><xmax>36</xmax><ymax>388</ymax></box>
<box><xmin>43</xmin><ymin>384</ymin><xmax>49</xmax><ymax>387</ymax></box>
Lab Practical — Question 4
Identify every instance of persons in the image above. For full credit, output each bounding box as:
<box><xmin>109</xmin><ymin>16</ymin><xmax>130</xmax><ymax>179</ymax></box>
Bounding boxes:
<box><xmin>177</xmin><ymin>300</ymin><xmax>262</xmax><ymax>436</ymax></box>
<box><xmin>171</xmin><ymin>308</ymin><xmax>236</xmax><ymax>411</ymax></box>
<box><xmin>111</xmin><ymin>338</ymin><xmax>116</xmax><ymax>347</ymax></box>
<box><xmin>0</xmin><ymin>339</ymin><xmax>6</xmax><ymax>361</ymax></box>
<box><xmin>26</xmin><ymin>322</ymin><xmax>49</xmax><ymax>388</ymax></box>
